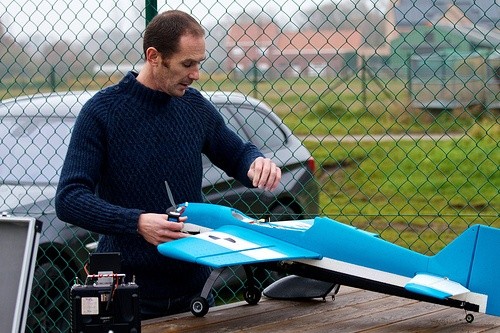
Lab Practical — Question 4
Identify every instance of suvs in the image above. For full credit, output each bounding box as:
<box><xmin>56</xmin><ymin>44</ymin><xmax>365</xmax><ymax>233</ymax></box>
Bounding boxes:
<box><xmin>0</xmin><ymin>86</ymin><xmax>323</xmax><ymax>332</ymax></box>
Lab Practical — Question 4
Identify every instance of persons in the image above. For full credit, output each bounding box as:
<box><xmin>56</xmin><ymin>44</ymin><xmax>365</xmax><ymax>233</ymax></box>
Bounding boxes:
<box><xmin>55</xmin><ymin>10</ymin><xmax>282</xmax><ymax>323</ymax></box>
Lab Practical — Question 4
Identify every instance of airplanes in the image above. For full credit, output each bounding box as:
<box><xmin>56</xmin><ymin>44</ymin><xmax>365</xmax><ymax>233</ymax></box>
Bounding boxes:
<box><xmin>157</xmin><ymin>200</ymin><xmax>500</xmax><ymax>324</ymax></box>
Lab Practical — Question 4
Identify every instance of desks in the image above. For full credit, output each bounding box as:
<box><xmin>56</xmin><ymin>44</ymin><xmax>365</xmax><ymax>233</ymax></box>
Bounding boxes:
<box><xmin>140</xmin><ymin>285</ymin><xmax>500</xmax><ymax>332</ymax></box>
